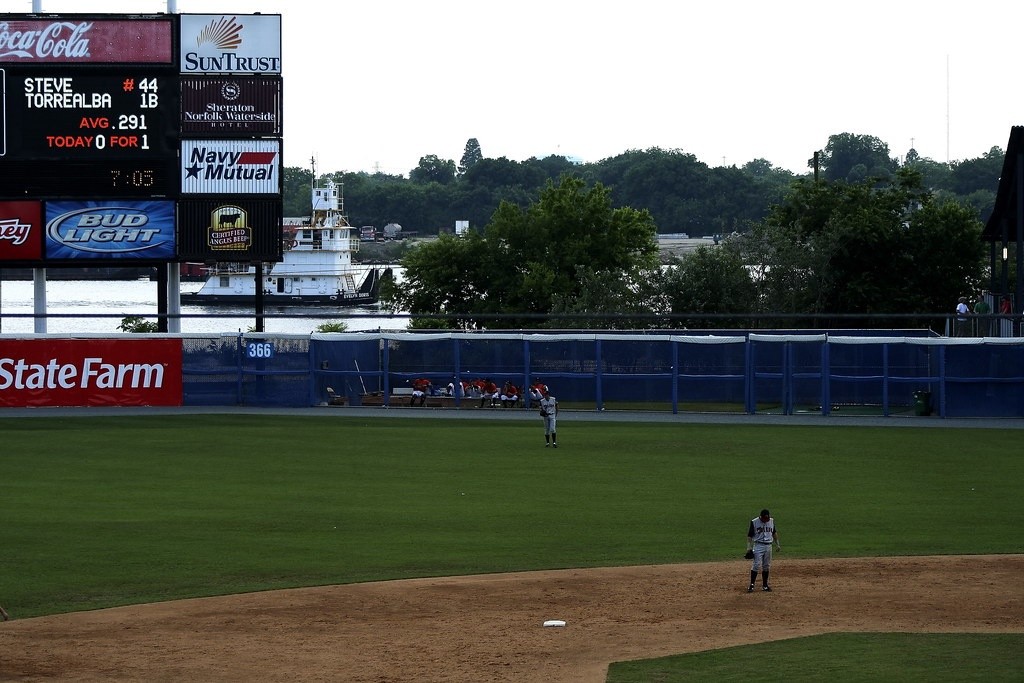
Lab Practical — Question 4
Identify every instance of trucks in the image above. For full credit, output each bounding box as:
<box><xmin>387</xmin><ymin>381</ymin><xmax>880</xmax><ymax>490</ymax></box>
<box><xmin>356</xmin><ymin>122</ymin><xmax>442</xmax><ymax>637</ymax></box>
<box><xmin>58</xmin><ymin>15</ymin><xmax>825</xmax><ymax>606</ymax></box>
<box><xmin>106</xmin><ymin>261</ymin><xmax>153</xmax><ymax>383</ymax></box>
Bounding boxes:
<box><xmin>359</xmin><ymin>226</ymin><xmax>377</xmax><ymax>242</ymax></box>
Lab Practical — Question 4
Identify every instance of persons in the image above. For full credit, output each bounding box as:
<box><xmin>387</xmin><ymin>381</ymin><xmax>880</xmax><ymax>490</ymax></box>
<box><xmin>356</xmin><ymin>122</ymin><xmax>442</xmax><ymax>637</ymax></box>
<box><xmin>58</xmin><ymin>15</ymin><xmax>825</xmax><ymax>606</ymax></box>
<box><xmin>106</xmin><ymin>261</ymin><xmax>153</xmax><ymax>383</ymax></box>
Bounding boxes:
<box><xmin>744</xmin><ymin>508</ymin><xmax>782</xmax><ymax>594</ymax></box>
<box><xmin>410</xmin><ymin>377</ymin><xmax>431</xmax><ymax>407</ymax></box>
<box><xmin>447</xmin><ymin>377</ymin><xmax>547</xmax><ymax>409</ymax></box>
<box><xmin>539</xmin><ymin>391</ymin><xmax>560</xmax><ymax>448</ymax></box>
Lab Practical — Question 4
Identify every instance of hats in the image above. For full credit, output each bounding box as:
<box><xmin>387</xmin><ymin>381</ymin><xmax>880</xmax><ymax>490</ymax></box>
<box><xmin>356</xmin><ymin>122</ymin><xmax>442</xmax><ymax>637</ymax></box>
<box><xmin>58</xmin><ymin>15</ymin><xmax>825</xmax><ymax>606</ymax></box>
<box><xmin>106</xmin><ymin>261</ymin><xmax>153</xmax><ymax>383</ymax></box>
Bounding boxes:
<box><xmin>761</xmin><ymin>509</ymin><xmax>770</xmax><ymax>522</ymax></box>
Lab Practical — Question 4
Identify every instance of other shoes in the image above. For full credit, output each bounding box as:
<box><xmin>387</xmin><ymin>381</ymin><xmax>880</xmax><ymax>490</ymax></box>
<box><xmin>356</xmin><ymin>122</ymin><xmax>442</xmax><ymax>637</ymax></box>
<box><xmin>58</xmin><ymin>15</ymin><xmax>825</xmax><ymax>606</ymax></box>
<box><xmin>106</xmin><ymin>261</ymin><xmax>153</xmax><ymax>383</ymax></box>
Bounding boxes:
<box><xmin>762</xmin><ymin>587</ymin><xmax>772</xmax><ymax>592</ymax></box>
<box><xmin>748</xmin><ymin>587</ymin><xmax>754</xmax><ymax>592</ymax></box>
<box><xmin>545</xmin><ymin>443</ymin><xmax>549</xmax><ymax>448</ymax></box>
<box><xmin>553</xmin><ymin>444</ymin><xmax>557</xmax><ymax>448</ymax></box>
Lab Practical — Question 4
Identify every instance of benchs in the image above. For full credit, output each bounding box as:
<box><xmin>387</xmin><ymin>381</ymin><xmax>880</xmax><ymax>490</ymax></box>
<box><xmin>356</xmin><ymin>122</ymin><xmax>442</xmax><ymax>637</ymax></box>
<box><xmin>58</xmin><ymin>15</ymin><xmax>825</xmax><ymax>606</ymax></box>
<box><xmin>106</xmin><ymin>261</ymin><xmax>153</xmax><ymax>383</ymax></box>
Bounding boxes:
<box><xmin>390</xmin><ymin>388</ymin><xmax>500</xmax><ymax>408</ymax></box>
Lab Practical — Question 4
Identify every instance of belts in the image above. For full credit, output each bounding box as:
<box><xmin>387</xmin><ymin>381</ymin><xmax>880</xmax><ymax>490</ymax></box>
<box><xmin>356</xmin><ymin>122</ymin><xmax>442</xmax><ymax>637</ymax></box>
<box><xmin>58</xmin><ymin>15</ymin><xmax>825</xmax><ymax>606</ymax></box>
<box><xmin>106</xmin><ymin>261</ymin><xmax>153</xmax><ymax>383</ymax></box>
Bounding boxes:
<box><xmin>756</xmin><ymin>541</ymin><xmax>772</xmax><ymax>545</ymax></box>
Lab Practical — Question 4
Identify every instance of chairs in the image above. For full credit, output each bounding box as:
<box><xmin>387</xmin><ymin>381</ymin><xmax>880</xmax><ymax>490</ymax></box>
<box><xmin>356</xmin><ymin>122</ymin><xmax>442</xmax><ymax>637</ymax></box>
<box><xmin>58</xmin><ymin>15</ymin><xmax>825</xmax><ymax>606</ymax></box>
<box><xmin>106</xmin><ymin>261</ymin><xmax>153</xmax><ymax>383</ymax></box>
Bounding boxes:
<box><xmin>507</xmin><ymin>385</ymin><xmax>523</xmax><ymax>408</ymax></box>
<box><xmin>326</xmin><ymin>386</ymin><xmax>342</xmax><ymax>406</ymax></box>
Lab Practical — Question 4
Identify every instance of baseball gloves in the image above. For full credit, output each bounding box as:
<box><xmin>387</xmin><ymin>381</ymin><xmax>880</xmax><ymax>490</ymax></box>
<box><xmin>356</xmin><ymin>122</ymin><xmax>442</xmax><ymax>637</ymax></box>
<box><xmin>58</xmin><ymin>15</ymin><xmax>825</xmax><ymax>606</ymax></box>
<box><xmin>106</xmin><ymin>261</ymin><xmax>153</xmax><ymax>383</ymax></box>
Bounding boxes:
<box><xmin>743</xmin><ymin>549</ymin><xmax>754</xmax><ymax>559</ymax></box>
<box><xmin>540</xmin><ymin>410</ymin><xmax>548</xmax><ymax>417</ymax></box>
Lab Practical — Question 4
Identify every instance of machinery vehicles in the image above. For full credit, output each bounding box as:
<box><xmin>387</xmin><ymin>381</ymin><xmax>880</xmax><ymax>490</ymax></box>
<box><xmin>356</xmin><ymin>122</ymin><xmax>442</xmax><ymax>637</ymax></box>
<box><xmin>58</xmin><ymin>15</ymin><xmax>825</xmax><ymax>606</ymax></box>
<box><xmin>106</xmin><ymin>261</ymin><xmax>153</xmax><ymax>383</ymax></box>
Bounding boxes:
<box><xmin>383</xmin><ymin>223</ymin><xmax>402</xmax><ymax>240</ymax></box>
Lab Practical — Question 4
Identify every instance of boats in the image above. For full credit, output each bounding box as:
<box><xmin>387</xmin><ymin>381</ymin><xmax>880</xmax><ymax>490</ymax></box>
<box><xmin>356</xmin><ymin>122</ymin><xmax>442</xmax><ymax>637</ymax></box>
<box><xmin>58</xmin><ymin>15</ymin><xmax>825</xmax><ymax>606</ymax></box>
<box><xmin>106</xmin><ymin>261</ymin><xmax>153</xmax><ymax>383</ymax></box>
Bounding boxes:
<box><xmin>179</xmin><ymin>156</ymin><xmax>393</xmax><ymax>307</ymax></box>
